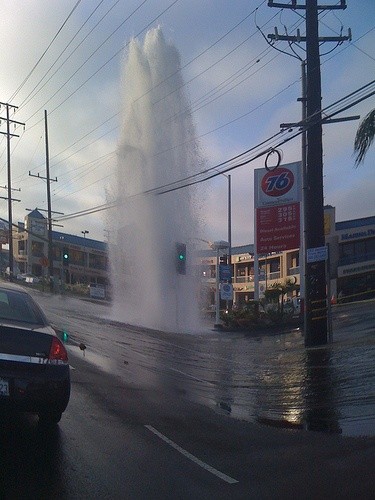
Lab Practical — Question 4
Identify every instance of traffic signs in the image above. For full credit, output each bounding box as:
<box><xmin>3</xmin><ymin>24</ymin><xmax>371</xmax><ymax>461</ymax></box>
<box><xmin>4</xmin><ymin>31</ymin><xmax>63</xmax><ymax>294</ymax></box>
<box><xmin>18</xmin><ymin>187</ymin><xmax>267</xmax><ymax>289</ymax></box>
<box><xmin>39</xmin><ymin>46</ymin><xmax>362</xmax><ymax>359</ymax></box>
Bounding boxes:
<box><xmin>220</xmin><ymin>283</ymin><xmax>234</xmax><ymax>301</ymax></box>
<box><xmin>219</xmin><ymin>262</ymin><xmax>234</xmax><ymax>281</ymax></box>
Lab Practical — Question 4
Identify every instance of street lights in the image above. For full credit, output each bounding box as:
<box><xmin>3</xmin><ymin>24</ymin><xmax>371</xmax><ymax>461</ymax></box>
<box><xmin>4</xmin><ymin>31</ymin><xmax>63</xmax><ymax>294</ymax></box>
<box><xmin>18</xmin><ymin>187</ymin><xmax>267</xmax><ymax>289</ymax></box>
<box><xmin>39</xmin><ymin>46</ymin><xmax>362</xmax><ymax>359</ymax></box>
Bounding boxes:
<box><xmin>81</xmin><ymin>230</ymin><xmax>89</xmax><ymax>283</ymax></box>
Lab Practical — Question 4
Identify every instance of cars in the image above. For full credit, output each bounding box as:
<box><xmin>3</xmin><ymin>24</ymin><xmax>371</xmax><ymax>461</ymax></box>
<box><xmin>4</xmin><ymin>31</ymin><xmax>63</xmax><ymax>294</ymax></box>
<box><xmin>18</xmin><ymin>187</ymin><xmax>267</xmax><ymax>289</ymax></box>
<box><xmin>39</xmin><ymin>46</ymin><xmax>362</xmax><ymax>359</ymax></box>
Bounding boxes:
<box><xmin>0</xmin><ymin>282</ymin><xmax>70</xmax><ymax>429</ymax></box>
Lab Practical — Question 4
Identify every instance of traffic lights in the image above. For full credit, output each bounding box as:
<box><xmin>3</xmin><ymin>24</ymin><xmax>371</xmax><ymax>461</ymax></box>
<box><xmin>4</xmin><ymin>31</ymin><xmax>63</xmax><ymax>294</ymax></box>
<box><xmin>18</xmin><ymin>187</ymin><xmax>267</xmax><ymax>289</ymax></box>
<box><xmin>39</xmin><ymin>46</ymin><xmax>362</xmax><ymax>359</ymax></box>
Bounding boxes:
<box><xmin>175</xmin><ymin>241</ymin><xmax>186</xmax><ymax>275</ymax></box>
